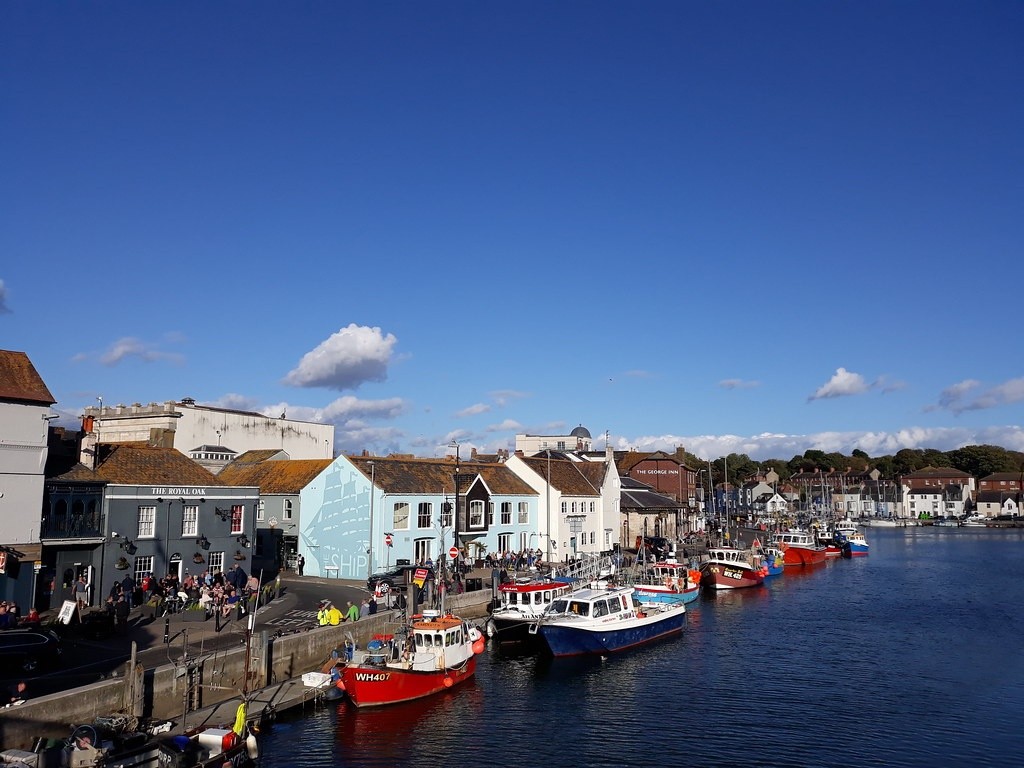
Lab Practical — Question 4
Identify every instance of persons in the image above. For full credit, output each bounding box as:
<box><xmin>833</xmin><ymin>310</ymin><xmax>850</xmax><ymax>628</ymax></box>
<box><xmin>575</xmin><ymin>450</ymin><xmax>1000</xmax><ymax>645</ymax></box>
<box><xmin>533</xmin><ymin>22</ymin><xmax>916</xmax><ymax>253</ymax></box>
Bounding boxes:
<box><xmin>0</xmin><ymin>600</ymin><xmax>39</xmax><ymax>629</ymax></box>
<box><xmin>491</xmin><ymin>566</ymin><xmax>500</xmax><ymax>587</ymax></box>
<box><xmin>464</xmin><ymin>554</ymin><xmax>471</xmax><ymax>574</ymax></box>
<box><xmin>111</xmin><ymin>563</ymin><xmax>259</xmax><ymax>616</ymax></box>
<box><xmin>297</xmin><ymin>553</ymin><xmax>305</xmax><ymax>577</ymax></box>
<box><xmin>569</xmin><ymin>556</ymin><xmax>582</xmax><ymax>576</ymax></box>
<box><xmin>316</xmin><ymin>602</ymin><xmax>343</xmax><ymax>627</ymax></box>
<box><xmin>345</xmin><ymin>601</ymin><xmax>360</xmax><ymax>621</ymax></box>
<box><xmin>360</xmin><ymin>597</ymin><xmax>377</xmax><ymax>616</ymax></box>
<box><xmin>392</xmin><ymin>590</ymin><xmax>406</xmax><ymax>610</ymax></box>
<box><xmin>72</xmin><ymin>577</ymin><xmax>86</xmax><ymax>610</ymax></box>
<box><xmin>500</xmin><ymin>566</ymin><xmax>507</xmax><ymax>584</ymax></box>
<box><xmin>426</xmin><ymin>558</ymin><xmax>432</xmax><ymax>565</ymax></box>
<box><xmin>11</xmin><ymin>682</ymin><xmax>26</xmax><ymax>702</ymax></box>
<box><xmin>486</xmin><ymin>548</ymin><xmax>543</xmax><ymax>569</ymax></box>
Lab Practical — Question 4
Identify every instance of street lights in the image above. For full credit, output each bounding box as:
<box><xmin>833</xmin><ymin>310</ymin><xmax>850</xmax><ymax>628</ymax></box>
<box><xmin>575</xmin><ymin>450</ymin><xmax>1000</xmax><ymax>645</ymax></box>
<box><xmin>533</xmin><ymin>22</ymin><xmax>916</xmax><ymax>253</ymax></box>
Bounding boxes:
<box><xmin>367</xmin><ymin>459</ymin><xmax>377</xmax><ymax>580</ymax></box>
<box><xmin>448</xmin><ymin>442</ymin><xmax>461</xmax><ymax>583</ymax></box>
<box><xmin>699</xmin><ymin>469</ymin><xmax>705</xmax><ymax>518</ymax></box>
<box><xmin>718</xmin><ymin>456</ymin><xmax>729</xmax><ymax>546</ymax></box>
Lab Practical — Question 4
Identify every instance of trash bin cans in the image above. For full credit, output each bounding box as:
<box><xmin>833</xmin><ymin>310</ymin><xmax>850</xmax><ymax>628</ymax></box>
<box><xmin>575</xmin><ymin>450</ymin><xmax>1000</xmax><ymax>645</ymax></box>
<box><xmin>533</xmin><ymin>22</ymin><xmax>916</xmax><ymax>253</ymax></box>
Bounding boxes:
<box><xmin>466</xmin><ymin>578</ymin><xmax>482</xmax><ymax>592</ymax></box>
<box><xmin>570</xmin><ymin>559</ymin><xmax>583</xmax><ymax>571</ymax></box>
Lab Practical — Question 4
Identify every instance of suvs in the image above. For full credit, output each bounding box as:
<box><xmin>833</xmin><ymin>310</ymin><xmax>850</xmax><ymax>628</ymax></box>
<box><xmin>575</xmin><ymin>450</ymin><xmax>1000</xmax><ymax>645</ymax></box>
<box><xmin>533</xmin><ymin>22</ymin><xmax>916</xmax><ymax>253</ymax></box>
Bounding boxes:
<box><xmin>366</xmin><ymin>564</ymin><xmax>436</xmax><ymax>594</ymax></box>
<box><xmin>0</xmin><ymin>626</ymin><xmax>79</xmax><ymax>702</ymax></box>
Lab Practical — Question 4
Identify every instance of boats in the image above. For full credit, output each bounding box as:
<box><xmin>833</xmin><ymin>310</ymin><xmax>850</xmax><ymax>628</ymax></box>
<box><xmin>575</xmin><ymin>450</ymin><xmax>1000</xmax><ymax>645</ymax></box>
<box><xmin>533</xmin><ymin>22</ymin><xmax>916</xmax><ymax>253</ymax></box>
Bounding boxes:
<box><xmin>694</xmin><ymin>469</ymin><xmax>869</xmax><ymax>590</ymax></box>
<box><xmin>960</xmin><ymin>518</ymin><xmax>986</xmax><ymax>527</ymax></box>
<box><xmin>631</xmin><ymin>560</ymin><xmax>702</xmax><ymax>607</ymax></box>
<box><xmin>488</xmin><ymin>577</ymin><xmax>594</xmax><ymax>644</ymax></box>
<box><xmin>291</xmin><ymin>484</ymin><xmax>486</xmax><ymax>708</ymax></box>
<box><xmin>13</xmin><ymin>607</ymin><xmax>265</xmax><ymax>768</ymax></box>
<box><xmin>526</xmin><ymin>585</ymin><xmax>687</xmax><ymax>657</ymax></box>
<box><xmin>932</xmin><ymin>517</ymin><xmax>961</xmax><ymax>527</ymax></box>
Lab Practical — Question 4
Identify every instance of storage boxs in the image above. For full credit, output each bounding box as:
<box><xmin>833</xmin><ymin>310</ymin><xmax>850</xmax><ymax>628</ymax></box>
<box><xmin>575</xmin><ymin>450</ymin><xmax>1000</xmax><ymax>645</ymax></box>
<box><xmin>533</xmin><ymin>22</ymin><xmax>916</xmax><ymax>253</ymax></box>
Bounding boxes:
<box><xmin>70</xmin><ymin>748</ymin><xmax>108</xmax><ymax>768</ymax></box>
<box><xmin>353</xmin><ymin>651</ymin><xmax>371</xmax><ymax>665</ymax></box>
<box><xmin>386</xmin><ymin>659</ymin><xmax>410</xmax><ymax>670</ymax></box>
<box><xmin>0</xmin><ymin>748</ymin><xmax>38</xmax><ymax>768</ymax></box>
<box><xmin>301</xmin><ymin>672</ymin><xmax>331</xmax><ymax>688</ymax></box>
<box><xmin>198</xmin><ymin>728</ymin><xmax>233</xmax><ymax>760</ymax></box>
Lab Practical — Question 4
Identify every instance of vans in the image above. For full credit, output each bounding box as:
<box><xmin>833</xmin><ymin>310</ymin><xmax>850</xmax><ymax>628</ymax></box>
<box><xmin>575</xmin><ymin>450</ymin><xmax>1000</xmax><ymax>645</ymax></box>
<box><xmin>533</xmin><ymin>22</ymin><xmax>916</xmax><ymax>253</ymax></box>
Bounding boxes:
<box><xmin>966</xmin><ymin>514</ymin><xmax>985</xmax><ymax>520</ymax></box>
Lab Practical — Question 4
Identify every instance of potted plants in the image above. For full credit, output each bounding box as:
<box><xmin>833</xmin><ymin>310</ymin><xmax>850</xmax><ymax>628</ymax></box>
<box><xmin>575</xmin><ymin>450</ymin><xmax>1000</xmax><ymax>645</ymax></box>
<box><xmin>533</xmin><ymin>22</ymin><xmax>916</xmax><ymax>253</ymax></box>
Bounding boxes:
<box><xmin>234</xmin><ymin>550</ymin><xmax>244</xmax><ymax>561</ymax></box>
<box><xmin>113</xmin><ymin>556</ymin><xmax>128</xmax><ymax>571</ymax></box>
<box><xmin>140</xmin><ymin>595</ymin><xmax>161</xmax><ymax>618</ymax></box>
<box><xmin>226</xmin><ymin>601</ymin><xmax>243</xmax><ymax>620</ymax></box>
<box><xmin>475</xmin><ymin>541</ymin><xmax>487</xmax><ymax>569</ymax></box>
<box><xmin>182</xmin><ymin>603</ymin><xmax>207</xmax><ymax>621</ymax></box>
<box><xmin>192</xmin><ymin>552</ymin><xmax>203</xmax><ymax>563</ymax></box>
<box><xmin>273</xmin><ymin>581</ymin><xmax>283</xmax><ymax>599</ymax></box>
<box><xmin>261</xmin><ymin>586</ymin><xmax>272</xmax><ymax>606</ymax></box>
<box><xmin>247</xmin><ymin>594</ymin><xmax>257</xmax><ymax>612</ymax></box>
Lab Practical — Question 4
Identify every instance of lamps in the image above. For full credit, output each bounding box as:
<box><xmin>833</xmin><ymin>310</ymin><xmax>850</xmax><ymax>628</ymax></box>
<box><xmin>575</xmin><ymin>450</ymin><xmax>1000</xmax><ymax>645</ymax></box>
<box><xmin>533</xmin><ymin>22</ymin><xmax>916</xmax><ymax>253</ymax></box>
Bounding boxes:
<box><xmin>196</xmin><ymin>533</ymin><xmax>207</xmax><ymax>546</ymax></box>
<box><xmin>42</xmin><ymin>413</ymin><xmax>59</xmax><ymax>421</ymax></box>
<box><xmin>179</xmin><ymin>498</ymin><xmax>185</xmax><ymax>504</ymax></box>
<box><xmin>215</xmin><ymin>506</ymin><xmax>235</xmax><ymax>522</ymax></box>
<box><xmin>236</xmin><ymin>532</ymin><xmax>247</xmax><ymax>547</ymax></box>
<box><xmin>112</xmin><ymin>536</ymin><xmax>131</xmax><ymax>551</ymax></box>
<box><xmin>199</xmin><ymin>498</ymin><xmax>206</xmax><ymax>503</ymax></box>
<box><xmin>156</xmin><ymin>498</ymin><xmax>163</xmax><ymax>503</ymax></box>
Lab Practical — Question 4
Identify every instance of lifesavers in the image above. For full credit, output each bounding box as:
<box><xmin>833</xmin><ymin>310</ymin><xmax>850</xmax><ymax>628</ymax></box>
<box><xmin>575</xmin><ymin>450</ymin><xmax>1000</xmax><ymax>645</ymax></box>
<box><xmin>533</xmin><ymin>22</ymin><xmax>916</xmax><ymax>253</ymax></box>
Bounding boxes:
<box><xmin>373</xmin><ymin>584</ymin><xmax>381</xmax><ymax>603</ymax></box>
<box><xmin>409</xmin><ymin>614</ymin><xmax>423</xmax><ymax>619</ymax></box>
<box><xmin>666</xmin><ymin>577</ymin><xmax>673</xmax><ymax>589</ymax></box>
<box><xmin>678</xmin><ymin>578</ymin><xmax>683</xmax><ymax>588</ymax></box>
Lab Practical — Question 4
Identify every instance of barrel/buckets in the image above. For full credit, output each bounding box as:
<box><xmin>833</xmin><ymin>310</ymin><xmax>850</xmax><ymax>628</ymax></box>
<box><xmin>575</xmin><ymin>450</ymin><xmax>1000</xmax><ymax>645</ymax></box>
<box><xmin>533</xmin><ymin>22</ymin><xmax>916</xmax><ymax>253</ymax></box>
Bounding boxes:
<box><xmin>173</xmin><ymin>736</ymin><xmax>189</xmax><ymax>750</ymax></box>
<box><xmin>372</xmin><ymin>633</ymin><xmax>394</xmax><ymax>641</ymax></box>
<box><xmin>367</xmin><ymin>640</ymin><xmax>383</xmax><ymax>663</ymax></box>
<box><xmin>32</xmin><ymin>737</ymin><xmax>48</xmax><ymax>754</ymax></box>
<box><xmin>331</xmin><ymin>667</ymin><xmax>339</xmax><ymax>681</ymax></box>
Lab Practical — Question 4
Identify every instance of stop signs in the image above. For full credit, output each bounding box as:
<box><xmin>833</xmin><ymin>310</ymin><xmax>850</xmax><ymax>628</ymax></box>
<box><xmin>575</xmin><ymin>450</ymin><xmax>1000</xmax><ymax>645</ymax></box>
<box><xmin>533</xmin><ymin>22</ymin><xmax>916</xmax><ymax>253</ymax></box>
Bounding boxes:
<box><xmin>449</xmin><ymin>547</ymin><xmax>458</xmax><ymax>558</ymax></box>
<box><xmin>386</xmin><ymin>537</ymin><xmax>392</xmax><ymax>545</ymax></box>
